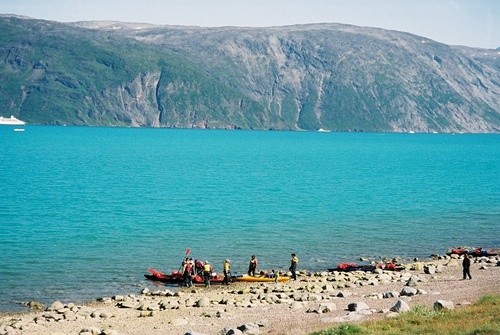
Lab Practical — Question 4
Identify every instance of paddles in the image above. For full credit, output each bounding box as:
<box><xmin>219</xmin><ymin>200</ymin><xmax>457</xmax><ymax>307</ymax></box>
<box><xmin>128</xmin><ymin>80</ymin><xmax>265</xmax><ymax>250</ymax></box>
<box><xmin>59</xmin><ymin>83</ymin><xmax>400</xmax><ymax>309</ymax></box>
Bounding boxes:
<box><xmin>177</xmin><ymin>249</ymin><xmax>190</xmax><ymax>274</ymax></box>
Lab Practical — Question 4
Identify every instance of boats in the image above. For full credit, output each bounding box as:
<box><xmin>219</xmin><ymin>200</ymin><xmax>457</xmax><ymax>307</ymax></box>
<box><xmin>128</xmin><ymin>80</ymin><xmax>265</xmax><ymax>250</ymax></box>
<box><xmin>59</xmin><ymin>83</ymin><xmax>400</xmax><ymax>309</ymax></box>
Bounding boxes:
<box><xmin>231</xmin><ymin>274</ymin><xmax>292</xmax><ymax>282</ymax></box>
<box><xmin>318</xmin><ymin>128</ymin><xmax>331</xmax><ymax>132</ymax></box>
<box><xmin>327</xmin><ymin>262</ymin><xmax>405</xmax><ymax>272</ymax></box>
<box><xmin>0</xmin><ymin>115</ymin><xmax>26</xmax><ymax>125</ymax></box>
<box><xmin>445</xmin><ymin>246</ymin><xmax>500</xmax><ymax>256</ymax></box>
<box><xmin>13</xmin><ymin>128</ymin><xmax>25</xmax><ymax>132</ymax></box>
<box><xmin>143</xmin><ymin>268</ymin><xmax>225</xmax><ymax>283</ymax></box>
<box><xmin>432</xmin><ymin>131</ymin><xmax>438</xmax><ymax>134</ymax></box>
<box><xmin>408</xmin><ymin>130</ymin><xmax>415</xmax><ymax>133</ymax></box>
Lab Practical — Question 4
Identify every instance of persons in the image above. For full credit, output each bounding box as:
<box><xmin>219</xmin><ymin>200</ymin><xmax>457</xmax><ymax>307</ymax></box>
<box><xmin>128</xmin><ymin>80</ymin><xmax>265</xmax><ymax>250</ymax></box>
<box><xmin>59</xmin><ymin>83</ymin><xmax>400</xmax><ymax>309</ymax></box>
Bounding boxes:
<box><xmin>181</xmin><ymin>258</ymin><xmax>205</xmax><ymax>287</ymax></box>
<box><xmin>288</xmin><ymin>253</ymin><xmax>298</xmax><ymax>281</ymax></box>
<box><xmin>461</xmin><ymin>252</ymin><xmax>472</xmax><ymax>279</ymax></box>
<box><xmin>222</xmin><ymin>259</ymin><xmax>231</xmax><ymax>284</ymax></box>
<box><xmin>247</xmin><ymin>255</ymin><xmax>258</xmax><ymax>276</ymax></box>
<box><xmin>204</xmin><ymin>261</ymin><xmax>213</xmax><ymax>287</ymax></box>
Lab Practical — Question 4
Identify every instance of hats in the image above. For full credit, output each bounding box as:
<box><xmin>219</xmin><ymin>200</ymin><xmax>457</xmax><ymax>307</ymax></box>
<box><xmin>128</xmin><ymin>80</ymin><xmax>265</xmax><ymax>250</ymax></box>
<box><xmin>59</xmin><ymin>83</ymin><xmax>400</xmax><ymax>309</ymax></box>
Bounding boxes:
<box><xmin>225</xmin><ymin>259</ymin><xmax>230</xmax><ymax>261</ymax></box>
<box><xmin>205</xmin><ymin>261</ymin><xmax>209</xmax><ymax>264</ymax></box>
<box><xmin>291</xmin><ymin>254</ymin><xmax>296</xmax><ymax>256</ymax></box>
